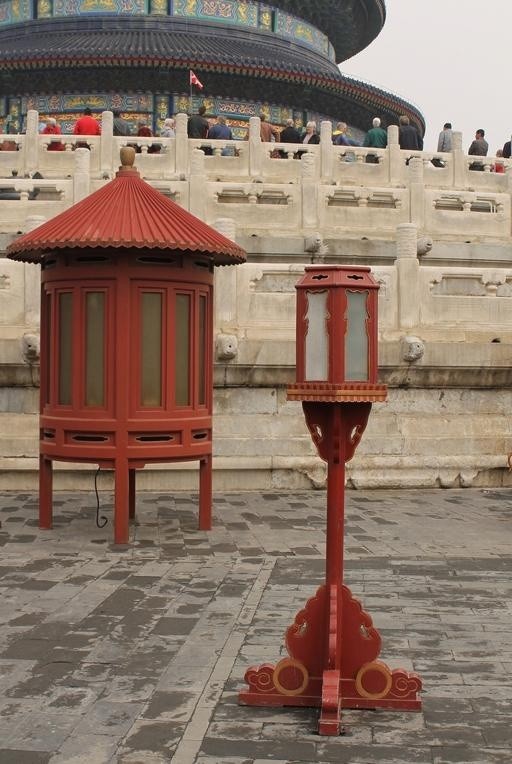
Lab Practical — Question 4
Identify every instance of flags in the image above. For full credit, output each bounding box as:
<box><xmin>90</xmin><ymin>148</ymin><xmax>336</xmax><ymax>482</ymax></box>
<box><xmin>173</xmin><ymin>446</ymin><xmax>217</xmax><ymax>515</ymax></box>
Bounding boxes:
<box><xmin>190</xmin><ymin>70</ymin><xmax>203</xmax><ymax>90</ymax></box>
